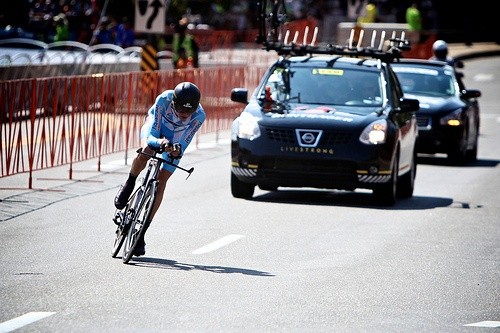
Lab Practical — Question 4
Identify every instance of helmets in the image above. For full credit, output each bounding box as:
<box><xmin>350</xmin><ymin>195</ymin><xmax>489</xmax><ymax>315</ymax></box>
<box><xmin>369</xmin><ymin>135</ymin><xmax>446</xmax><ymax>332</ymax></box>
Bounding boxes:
<box><xmin>171</xmin><ymin>82</ymin><xmax>201</xmax><ymax>119</ymax></box>
<box><xmin>432</xmin><ymin>40</ymin><xmax>449</xmax><ymax>57</ymax></box>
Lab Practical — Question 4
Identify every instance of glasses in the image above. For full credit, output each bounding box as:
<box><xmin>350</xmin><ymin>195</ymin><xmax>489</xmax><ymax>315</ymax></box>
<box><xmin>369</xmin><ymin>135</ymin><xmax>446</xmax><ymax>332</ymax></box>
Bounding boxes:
<box><xmin>174</xmin><ymin>106</ymin><xmax>194</xmax><ymax>114</ymax></box>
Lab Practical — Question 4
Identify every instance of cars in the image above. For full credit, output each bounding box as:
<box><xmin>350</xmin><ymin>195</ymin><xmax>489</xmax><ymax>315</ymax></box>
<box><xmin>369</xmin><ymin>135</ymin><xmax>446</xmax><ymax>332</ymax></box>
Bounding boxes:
<box><xmin>391</xmin><ymin>58</ymin><xmax>481</xmax><ymax>166</ymax></box>
<box><xmin>230</xmin><ymin>54</ymin><xmax>420</xmax><ymax>208</ymax></box>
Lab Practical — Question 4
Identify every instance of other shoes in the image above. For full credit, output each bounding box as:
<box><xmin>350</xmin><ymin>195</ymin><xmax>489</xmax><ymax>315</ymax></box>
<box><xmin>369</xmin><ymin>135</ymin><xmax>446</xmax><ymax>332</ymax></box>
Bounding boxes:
<box><xmin>132</xmin><ymin>220</ymin><xmax>151</xmax><ymax>256</ymax></box>
<box><xmin>114</xmin><ymin>173</ymin><xmax>137</xmax><ymax>210</ymax></box>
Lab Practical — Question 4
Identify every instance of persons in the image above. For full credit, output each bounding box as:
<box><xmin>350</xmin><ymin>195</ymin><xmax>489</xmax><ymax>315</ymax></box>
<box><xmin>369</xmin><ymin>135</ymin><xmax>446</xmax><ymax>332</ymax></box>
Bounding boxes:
<box><xmin>427</xmin><ymin>39</ymin><xmax>454</xmax><ymax>82</ymax></box>
<box><xmin>115</xmin><ymin>81</ymin><xmax>206</xmax><ymax>255</ymax></box>
<box><xmin>352</xmin><ymin>74</ymin><xmax>380</xmax><ymax>103</ymax></box>
<box><xmin>0</xmin><ymin>0</ymin><xmax>421</xmax><ymax>68</ymax></box>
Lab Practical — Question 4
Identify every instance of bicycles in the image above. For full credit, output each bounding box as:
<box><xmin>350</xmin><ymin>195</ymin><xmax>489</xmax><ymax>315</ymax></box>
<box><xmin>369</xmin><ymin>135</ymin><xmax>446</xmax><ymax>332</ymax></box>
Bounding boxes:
<box><xmin>111</xmin><ymin>147</ymin><xmax>194</xmax><ymax>264</ymax></box>
